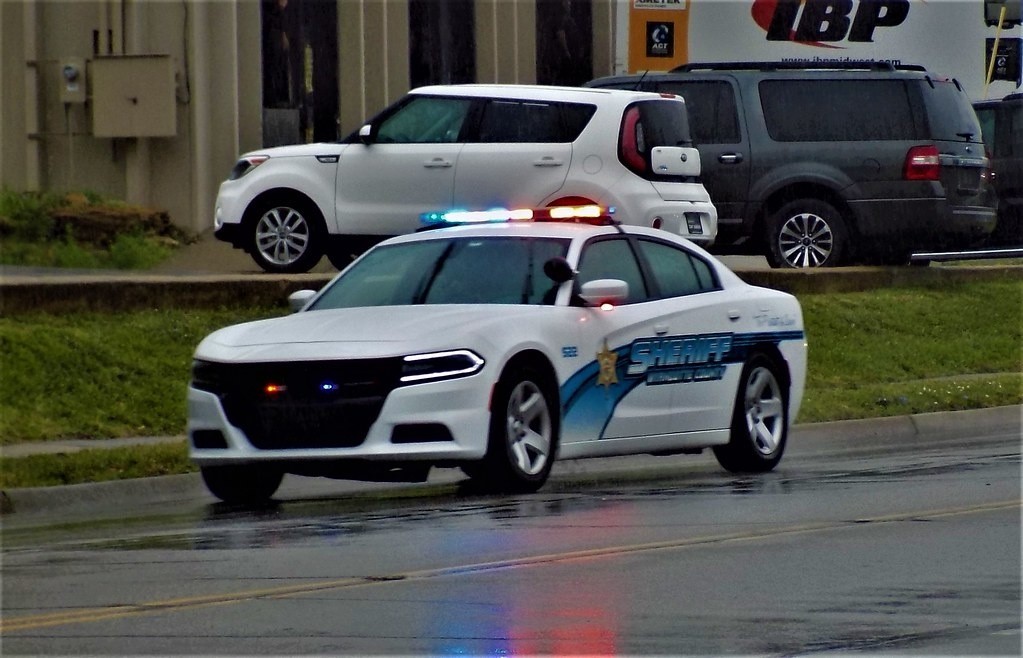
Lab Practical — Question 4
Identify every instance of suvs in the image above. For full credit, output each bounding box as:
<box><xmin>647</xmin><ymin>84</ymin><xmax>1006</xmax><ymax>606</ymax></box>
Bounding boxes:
<box><xmin>213</xmin><ymin>83</ymin><xmax>718</xmax><ymax>274</ymax></box>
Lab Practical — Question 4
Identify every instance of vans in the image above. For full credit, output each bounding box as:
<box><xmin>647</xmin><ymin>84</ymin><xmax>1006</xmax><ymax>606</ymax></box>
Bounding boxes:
<box><xmin>971</xmin><ymin>93</ymin><xmax>1023</xmax><ymax>246</ymax></box>
<box><xmin>580</xmin><ymin>61</ymin><xmax>997</xmax><ymax>269</ymax></box>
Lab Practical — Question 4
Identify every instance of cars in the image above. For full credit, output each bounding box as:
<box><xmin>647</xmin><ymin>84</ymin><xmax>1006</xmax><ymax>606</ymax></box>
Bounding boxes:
<box><xmin>186</xmin><ymin>206</ymin><xmax>808</xmax><ymax>502</ymax></box>
